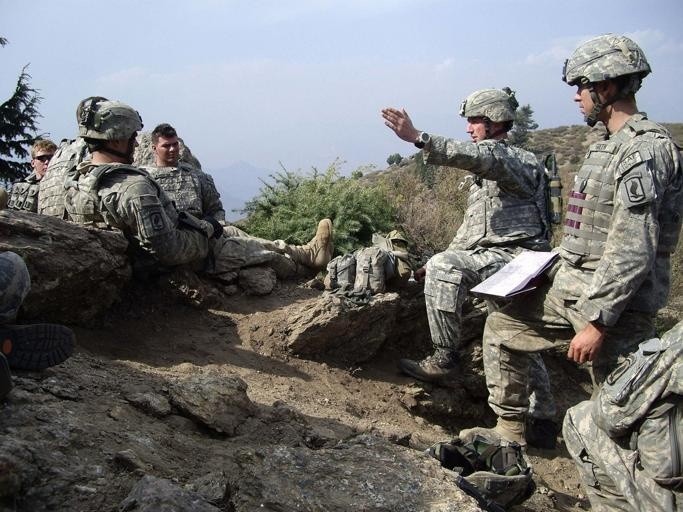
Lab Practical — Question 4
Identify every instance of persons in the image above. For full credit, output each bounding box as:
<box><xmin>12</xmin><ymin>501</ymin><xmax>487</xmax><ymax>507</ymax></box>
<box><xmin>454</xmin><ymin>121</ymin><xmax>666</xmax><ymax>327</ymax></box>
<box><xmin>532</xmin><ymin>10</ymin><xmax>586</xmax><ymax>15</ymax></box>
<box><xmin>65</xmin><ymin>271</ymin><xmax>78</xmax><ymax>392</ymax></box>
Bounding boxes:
<box><xmin>36</xmin><ymin>97</ymin><xmax>112</xmax><ymax>221</ymax></box>
<box><xmin>133</xmin><ymin>123</ymin><xmax>243</xmax><ymax>292</ymax></box>
<box><xmin>0</xmin><ymin>250</ymin><xmax>78</xmax><ymax>404</ymax></box>
<box><xmin>559</xmin><ymin>319</ymin><xmax>683</xmax><ymax>511</ymax></box>
<box><xmin>0</xmin><ymin>139</ymin><xmax>60</xmax><ymax>214</ymax></box>
<box><xmin>460</xmin><ymin>33</ymin><xmax>682</xmax><ymax>455</ymax></box>
<box><xmin>62</xmin><ymin>98</ymin><xmax>333</xmax><ymax>282</ymax></box>
<box><xmin>378</xmin><ymin>88</ymin><xmax>556</xmax><ymax>389</ymax></box>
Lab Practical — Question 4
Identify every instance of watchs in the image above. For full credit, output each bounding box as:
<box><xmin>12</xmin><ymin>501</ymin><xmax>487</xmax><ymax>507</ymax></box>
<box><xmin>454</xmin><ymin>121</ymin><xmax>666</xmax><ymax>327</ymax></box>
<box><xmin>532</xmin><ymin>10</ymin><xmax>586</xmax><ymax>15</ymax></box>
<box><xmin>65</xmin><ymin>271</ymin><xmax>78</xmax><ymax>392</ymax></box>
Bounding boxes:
<box><xmin>414</xmin><ymin>131</ymin><xmax>430</xmax><ymax>149</ymax></box>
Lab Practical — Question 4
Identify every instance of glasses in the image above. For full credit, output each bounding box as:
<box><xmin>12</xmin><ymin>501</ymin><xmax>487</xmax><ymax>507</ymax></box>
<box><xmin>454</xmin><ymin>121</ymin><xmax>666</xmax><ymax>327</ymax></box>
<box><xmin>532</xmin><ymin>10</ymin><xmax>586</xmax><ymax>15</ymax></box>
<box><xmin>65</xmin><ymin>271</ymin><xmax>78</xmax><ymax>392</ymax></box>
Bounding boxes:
<box><xmin>33</xmin><ymin>155</ymin><xmax>52</xmax><ymax>161</ymax></box>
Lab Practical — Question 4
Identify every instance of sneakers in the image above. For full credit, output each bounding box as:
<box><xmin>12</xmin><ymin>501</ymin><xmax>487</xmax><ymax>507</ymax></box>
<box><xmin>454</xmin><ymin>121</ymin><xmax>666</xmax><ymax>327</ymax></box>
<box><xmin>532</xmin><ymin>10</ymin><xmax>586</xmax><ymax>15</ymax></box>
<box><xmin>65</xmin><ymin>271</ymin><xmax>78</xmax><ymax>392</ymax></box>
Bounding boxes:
<box><xmin>0</xmin><ymin>324</ymin><xmax>78</xmax><ymax>371</ymax></box>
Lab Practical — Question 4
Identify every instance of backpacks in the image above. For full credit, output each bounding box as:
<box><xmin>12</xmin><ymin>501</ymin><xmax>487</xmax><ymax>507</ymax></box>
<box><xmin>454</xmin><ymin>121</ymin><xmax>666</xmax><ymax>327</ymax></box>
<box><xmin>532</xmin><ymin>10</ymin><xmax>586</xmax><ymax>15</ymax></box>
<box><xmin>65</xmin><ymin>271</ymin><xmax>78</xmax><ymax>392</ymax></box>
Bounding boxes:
<box><xmin>324</xmin><ymin>230</ymin><xmax>413</xmax><ymax>303</ymax></box>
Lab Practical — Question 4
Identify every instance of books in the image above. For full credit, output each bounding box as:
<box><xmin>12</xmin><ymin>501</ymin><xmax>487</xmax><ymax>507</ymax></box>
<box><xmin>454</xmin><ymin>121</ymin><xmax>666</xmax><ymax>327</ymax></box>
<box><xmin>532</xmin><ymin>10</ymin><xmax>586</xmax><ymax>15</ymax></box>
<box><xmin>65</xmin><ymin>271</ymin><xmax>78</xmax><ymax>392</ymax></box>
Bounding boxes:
<box><xmin>468</xmin><ymin>250</ymin><xmax>561</xmax><ymax>297</ymax></box>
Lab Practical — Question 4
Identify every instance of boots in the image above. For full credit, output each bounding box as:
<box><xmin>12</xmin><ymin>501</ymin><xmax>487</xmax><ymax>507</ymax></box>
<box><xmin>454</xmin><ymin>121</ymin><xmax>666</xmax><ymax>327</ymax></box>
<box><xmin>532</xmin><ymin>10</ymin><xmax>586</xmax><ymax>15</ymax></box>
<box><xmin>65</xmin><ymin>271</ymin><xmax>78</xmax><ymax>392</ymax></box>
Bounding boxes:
<box><xmin>527</xmin><ymin>417</ymin><xmax>558</xmax><ymax>450</ymax></box>
<box><xmin>285</xmin><ymin>218</ymin><xmax>333</xmax><ymax>267</ymax></box>
<box><xmin>400</xmin><ymin>348</ymin><xmax>464</xmax><ymax>385</ymax></box>
<box><xmin>458</xmin><ymin>418</ymin><xmax>528</xmax><ymax>454</ymax></box>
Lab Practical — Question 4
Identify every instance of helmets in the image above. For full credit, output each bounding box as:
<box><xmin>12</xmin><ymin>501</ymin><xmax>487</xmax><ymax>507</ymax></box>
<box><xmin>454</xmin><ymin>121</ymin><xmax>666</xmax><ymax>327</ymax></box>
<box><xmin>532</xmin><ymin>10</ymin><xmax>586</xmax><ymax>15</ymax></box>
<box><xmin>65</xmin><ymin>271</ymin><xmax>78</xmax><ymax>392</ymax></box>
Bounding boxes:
<box><xmin>559</xmin><ymin>32</ymin><xmax>652</xmax><ymax>84</ymax></box>
<box><xmin>75</xmin><ymin>95</ymin><xmax>143</xmax><ymax>141</ymax></box>
<box><xmin>457</xmin><ymin>86</ymin><xmax>522</xmax><ymax>124</ymax></box>
